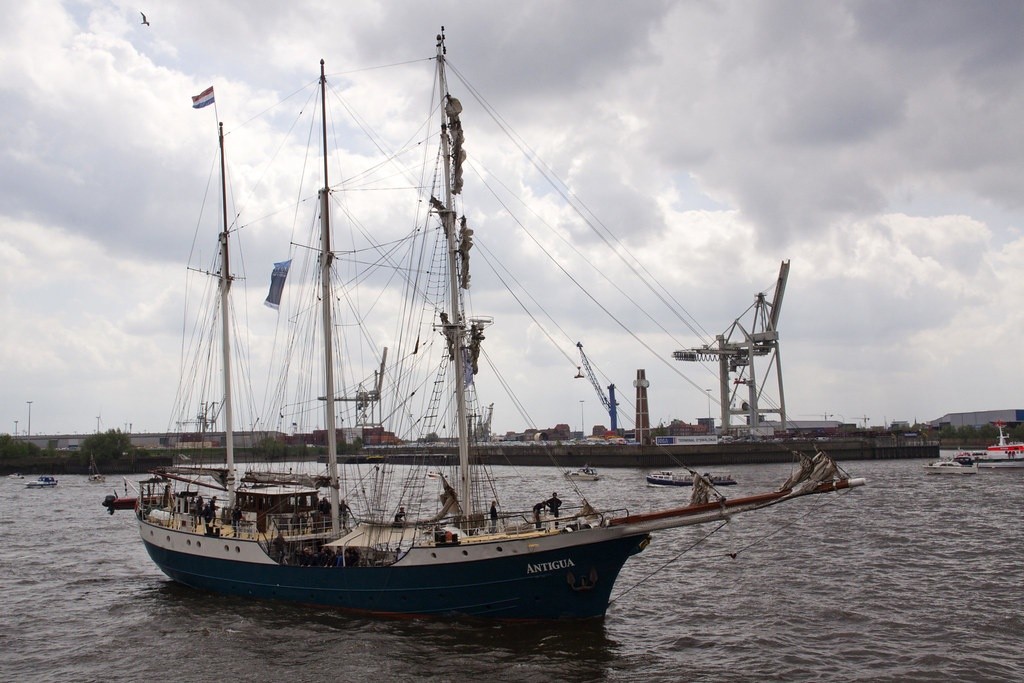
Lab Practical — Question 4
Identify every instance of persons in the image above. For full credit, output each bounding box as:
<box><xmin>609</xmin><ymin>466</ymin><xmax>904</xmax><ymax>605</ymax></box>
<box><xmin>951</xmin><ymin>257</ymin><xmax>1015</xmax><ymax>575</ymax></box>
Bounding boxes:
<box><xmin>314</xmin><ymin>497</ymin><xmax>332</xmax><ymax>530</ymax></box>
<box><xmin>210</xmin><ymin>496</ymin><xmax>218</xmax><ymax>527</ymax></box>
<box><xmin>1008</xmin><ymin>451</ymin><xmax>1015</xmax><ymax>459</ymax></box>
<box><xmin>231</xmin><ymin>505</ymin><xmax>242</xmax><ymax>537</ymax></box>
<box><xmin>490</xmin><ymin>501</ymin><xmax>498</xmax><ymax>530</ymax></box>
<box><xmin>533</xmin><ymin>501</ymin><xmax>546</xmax><ymax>528</ymax></box>
<box><xmin>395</xmin><ymin>507</ymin><xmax>405</xmax><ymax>522</ymax></box>
<box><xmin>201</xmin><ymin>503</ymin><xmax>212</xmax><ymax>532</ymax></box>
<box><xmin>546</xmin><ymin>492</ymin><xmax>562</xmax><ymax>529</ymax></box>
<box><xmin>196</xmin><ymin>496</ymin><xmax>203</xmax><ymax>524</ymax></box>
<box><xmin>340</xmin><ymin>500</ymin><xmax>350</xmax><ymax>528</ymax></box>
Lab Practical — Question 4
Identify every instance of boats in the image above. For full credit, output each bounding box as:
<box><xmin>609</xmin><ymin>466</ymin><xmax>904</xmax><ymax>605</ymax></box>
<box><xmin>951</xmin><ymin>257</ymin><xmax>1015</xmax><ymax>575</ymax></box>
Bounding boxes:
<box><xmin>922</xmin><ymin>448</ymin><xmax>980</xmax><ymax>474</ymax></box>
<box><xmin>951</xmin><ymin>420</ymin><xmax>1024</xmax><ymax>468</ymax></box>
<box><xmin>563</xmin><ymin>464</ymin><xmax>602</xmax><ymax>481</ymax></box>
<box><xmin>645</xmin><ymin>470</ymin><xmax>738</xmax><ymax>487</ymax></box>
<box><xmin>9</xmin><ymin>473</ymin><xmax>25</xmax><ymax>479</ymax></box>
<box><xmin>22</xmin><ymin>474</ymin><xmax>58</xmax><ymax>488</ymax></box>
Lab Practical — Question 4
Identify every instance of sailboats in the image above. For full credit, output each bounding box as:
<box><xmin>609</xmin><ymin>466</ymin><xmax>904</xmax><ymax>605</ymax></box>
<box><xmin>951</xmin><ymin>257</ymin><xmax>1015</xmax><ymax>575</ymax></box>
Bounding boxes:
<box><xmin>87</xmin><ymin>449</ymin><xmax>105</xmax><ymax>484</ymax></box>
<box><xmin>102</xmin><ymin>24</ymin><xmax>654</xmax><ymax>624</ymax></box>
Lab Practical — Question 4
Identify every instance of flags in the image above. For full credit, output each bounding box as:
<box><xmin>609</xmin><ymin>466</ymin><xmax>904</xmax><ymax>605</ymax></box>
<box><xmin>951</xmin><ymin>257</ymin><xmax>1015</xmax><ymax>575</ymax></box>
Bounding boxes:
<box><xmin>192</xmin><ymin>86</ymin><xmax>215</xmax><ymax>109</ymax></box>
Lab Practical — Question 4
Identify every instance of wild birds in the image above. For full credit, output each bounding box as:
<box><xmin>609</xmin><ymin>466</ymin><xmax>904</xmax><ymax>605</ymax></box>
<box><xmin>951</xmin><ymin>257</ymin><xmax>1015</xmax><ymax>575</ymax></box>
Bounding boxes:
<box><xmin>140</xmin><ymin>12</ymin><xmax>149</xmax><ymax>26</ymax></box>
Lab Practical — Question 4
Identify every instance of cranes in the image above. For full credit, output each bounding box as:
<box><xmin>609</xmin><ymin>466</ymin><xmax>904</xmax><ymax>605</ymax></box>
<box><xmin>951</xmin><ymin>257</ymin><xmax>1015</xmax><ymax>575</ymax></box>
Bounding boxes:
<box><xmin>576</xmin><ymin>341</ymin><xmax>620</xmax><ymax>431</ymax></box>
<box><xmin>670</xmin><ymin>257</ymin><xmax>790</xmax><ymax>435</ymax></box>
<box><xmin>317</xmin><ymin>346</ymin><xmax>388</xmax><ymax>427</ymax></box>
